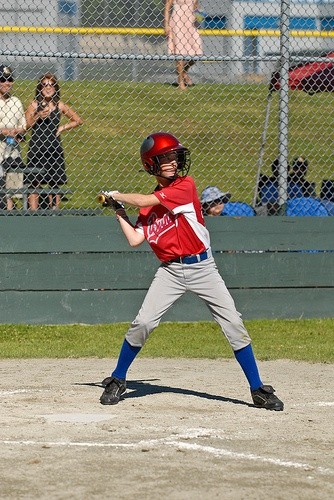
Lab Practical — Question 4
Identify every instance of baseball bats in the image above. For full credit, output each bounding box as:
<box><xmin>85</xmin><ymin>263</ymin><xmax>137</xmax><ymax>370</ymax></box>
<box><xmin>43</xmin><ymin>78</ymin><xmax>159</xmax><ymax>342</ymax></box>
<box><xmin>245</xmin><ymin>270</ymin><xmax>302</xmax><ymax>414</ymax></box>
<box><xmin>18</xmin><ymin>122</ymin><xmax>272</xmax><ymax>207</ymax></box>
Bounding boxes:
<box><xmin>98</xmin><ymin>193</ymin><xmax>118</xmax><ymax>206</ymax></box>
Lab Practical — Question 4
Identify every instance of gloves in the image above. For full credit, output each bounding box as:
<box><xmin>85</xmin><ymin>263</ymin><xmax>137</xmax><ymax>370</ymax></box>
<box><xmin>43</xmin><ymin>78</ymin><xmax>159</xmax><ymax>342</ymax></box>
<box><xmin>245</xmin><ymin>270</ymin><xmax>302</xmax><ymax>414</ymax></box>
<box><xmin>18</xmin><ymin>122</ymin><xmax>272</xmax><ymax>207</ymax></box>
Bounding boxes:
<box><xmin>101</xmin><ymin>190</ymin><xmax>125</xmax><ymax>213</ymax></box>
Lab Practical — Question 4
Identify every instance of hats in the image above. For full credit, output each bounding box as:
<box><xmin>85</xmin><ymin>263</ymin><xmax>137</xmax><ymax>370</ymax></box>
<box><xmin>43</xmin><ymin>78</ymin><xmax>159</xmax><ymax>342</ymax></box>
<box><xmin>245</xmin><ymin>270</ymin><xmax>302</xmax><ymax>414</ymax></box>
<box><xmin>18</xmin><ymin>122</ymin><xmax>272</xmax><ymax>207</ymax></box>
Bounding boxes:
<box><xmin>200</xmin><ymin>186</ymin><xmax>231</xmax><ymax>208</ymax></box>
<box><xmin>0</xmin><ymin>65</ymin><xmax>13</xmax><ymax>76</ymax></box>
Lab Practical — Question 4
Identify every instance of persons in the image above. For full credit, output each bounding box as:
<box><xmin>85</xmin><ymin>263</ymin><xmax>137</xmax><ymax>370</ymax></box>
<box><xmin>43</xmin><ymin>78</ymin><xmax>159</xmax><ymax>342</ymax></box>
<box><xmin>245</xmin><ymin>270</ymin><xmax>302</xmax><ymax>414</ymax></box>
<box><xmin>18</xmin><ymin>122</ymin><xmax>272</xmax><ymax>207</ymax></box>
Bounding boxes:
<box><xmin>165</xmin><ymin>0</ymin><xmax>203</xmax><ymax>91</ymax></box>
<box><xmin>100</xmin><ymin>133</ymin><xmax>285</xmax><ymax>411</ymax></box>
<box><xmin>200</xmin><ymin>156</ymin><xmax>333</xmax><ymax>216</ymax></box>
<box><xmin>26</xmin><ymin>74</ymin><xmax>82</xmax><ymax>210</ymax></box>
<box><xmin>0</xmin><ymin>65</ymin><xmax>27</xmax><ymax>210</ymax></box>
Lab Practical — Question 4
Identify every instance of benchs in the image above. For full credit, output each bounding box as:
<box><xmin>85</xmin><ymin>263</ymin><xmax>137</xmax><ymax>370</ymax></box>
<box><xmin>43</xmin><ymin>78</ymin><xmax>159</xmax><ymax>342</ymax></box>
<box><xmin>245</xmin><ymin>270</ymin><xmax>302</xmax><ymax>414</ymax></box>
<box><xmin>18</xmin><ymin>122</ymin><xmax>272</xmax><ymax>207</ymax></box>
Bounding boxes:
<box><xmin>0</xmin><ymin>210</ymin><xmax>103</xmax><ymax>217</ymax></box>
<box><xmin>0</xmin><ymin>189</ymin><xmax>72</xmax><ymax>210</ymax></box>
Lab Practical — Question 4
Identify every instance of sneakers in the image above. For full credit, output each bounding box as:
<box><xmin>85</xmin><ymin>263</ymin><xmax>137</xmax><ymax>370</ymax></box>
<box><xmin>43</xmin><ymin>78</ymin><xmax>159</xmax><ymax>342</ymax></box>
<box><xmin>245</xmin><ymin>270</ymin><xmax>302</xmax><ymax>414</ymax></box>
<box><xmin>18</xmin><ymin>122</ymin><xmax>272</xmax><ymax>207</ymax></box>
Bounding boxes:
<box><xmin>99</xmin><ymin>377</ymin><xmax>126</xmax><ymax>405</ymax></box>
<box><xmin>250</xmin><ymin>385</ymin><xmax>283</xmax><ymax>411</ymax></box>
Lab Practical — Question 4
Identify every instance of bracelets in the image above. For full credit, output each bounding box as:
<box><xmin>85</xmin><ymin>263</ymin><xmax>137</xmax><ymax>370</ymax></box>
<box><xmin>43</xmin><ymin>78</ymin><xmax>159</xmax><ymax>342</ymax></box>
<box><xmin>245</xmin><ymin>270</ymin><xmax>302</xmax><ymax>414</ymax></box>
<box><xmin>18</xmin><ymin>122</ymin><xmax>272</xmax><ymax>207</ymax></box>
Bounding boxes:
<box><xmin>116</xmin><ymin>215</ymin><xmax>129</xmax><ymax>221</ymax></box>
<box><xmin>63</xmin><ymin>125</ymin><xmax>66</xmax><ymax>131</ymax></box>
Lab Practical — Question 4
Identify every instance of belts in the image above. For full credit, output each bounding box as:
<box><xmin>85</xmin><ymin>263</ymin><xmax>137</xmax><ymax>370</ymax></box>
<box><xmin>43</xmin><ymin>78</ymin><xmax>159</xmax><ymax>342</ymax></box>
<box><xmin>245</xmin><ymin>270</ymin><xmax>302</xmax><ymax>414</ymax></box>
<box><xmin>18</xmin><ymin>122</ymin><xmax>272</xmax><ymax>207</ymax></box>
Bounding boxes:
<box><xmin>175</xmin><ymin>246</ymin><xmax>212</xmax><ymax>264</ymax></box>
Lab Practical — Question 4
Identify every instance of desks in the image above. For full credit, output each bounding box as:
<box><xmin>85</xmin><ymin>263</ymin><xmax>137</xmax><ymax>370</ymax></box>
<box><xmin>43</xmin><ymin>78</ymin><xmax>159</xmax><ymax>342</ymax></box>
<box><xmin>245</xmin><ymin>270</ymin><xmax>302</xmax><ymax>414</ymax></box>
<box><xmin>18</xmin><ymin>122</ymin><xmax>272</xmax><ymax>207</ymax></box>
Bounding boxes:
<box><xmin>7</xmin><ymin>167</ymin><xmax>45</xmax><ymax>209</ymax></box>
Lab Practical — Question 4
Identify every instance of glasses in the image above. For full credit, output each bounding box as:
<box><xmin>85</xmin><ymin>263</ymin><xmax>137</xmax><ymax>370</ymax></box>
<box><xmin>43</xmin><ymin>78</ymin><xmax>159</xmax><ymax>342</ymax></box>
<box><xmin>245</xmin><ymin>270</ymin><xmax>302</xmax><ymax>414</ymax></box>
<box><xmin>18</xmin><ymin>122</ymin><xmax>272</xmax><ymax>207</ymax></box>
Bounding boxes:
<box><xmin>0</xmin><ymin>76</ymin><xmax>13</xmax><ymax>82</ymax></box>
<box><xmin>41</xmin><ymin>83</ymin><xmax>56</xmax><ymax>88</ymax></box>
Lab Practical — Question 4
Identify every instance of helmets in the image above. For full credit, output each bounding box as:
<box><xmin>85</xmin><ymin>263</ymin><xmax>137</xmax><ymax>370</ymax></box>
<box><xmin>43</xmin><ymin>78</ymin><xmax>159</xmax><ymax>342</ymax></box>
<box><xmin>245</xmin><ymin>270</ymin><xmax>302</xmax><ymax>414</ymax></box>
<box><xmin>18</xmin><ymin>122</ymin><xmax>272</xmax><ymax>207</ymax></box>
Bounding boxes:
<box><xmin>140</xmin><ymin>132</ymin><xmax>191</xmax><ymax>181</ymax></box>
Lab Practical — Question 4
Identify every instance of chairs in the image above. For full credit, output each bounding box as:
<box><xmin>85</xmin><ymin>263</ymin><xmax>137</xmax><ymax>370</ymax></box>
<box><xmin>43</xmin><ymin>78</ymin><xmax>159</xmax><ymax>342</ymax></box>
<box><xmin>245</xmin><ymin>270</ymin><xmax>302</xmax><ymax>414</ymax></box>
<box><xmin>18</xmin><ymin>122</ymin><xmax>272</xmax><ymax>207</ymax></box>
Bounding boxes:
<box><xmin>222</xmin><ymin>202</ymin><xmax>256</xmax><ymax>216</ymax></box>
<box><xmin>286</xmin><ymin>198</ymin><xmax>330</xmax><ymax>253</ymax></box>
<box><xmin>262</xmin><ymin>183</ymin><xmax>303</xmax><ymax>206</ymax></box>
